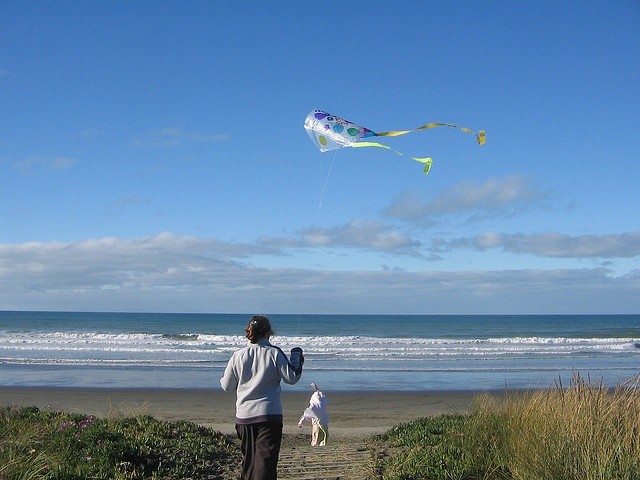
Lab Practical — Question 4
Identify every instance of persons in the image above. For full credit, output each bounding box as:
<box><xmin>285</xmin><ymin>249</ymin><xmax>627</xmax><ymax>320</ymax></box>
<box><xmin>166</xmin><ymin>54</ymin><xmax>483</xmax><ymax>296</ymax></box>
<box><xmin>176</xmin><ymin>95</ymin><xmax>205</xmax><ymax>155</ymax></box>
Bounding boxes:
<box><xmin>217</xmin><ymin>315</ymin><xmax>304</xmax><ymax>480</ymax></box>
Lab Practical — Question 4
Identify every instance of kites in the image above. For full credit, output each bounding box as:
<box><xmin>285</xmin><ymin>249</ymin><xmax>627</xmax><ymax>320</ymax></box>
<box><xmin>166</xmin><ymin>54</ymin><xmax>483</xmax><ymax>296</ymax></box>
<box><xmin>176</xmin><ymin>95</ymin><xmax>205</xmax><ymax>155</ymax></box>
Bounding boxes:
<box><xmin>303</xmin><ymin>107</ymin><xmax>486</xmax><ymax>176</ymax></box>
